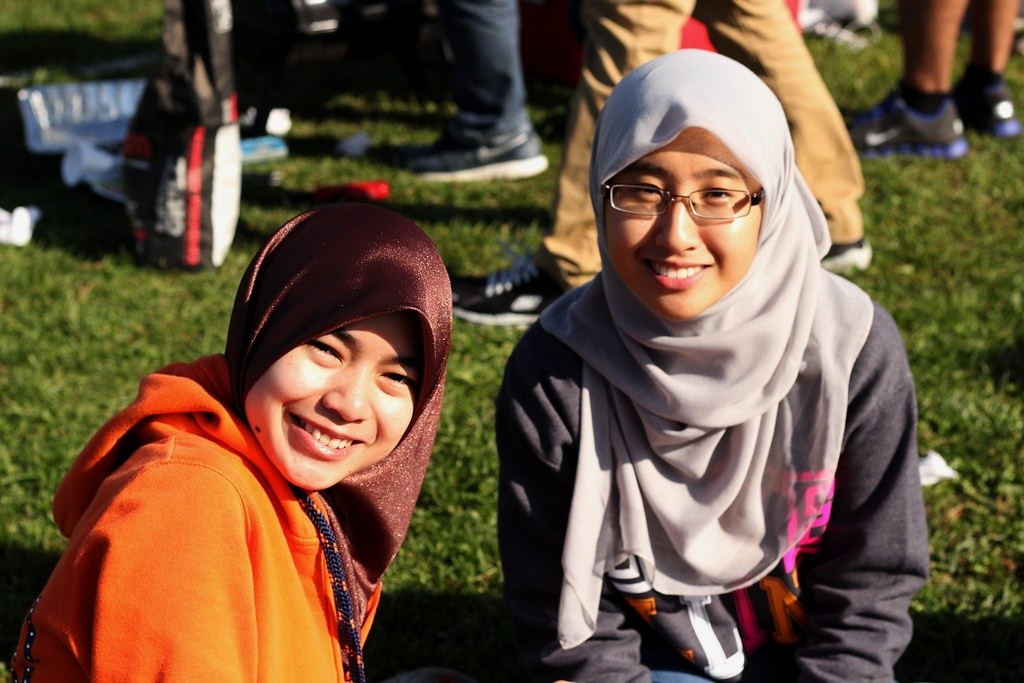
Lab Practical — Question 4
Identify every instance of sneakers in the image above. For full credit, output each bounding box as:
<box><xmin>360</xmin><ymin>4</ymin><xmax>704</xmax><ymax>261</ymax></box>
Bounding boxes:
<box><xmin>841</xmin><ymin>90</ymin><xmax>968</xmax><ymax>158</ymax></box>
<box><xmin>448</xmin><ymin>241</ymin><xmax>564</xmax><ymax>325</ymax></box>
<box><xmin>820</xmin><ymin>240</ymin><xmax>872</xmax><ymax>272</ymax></box>
<box><xmin>409</xmin><ymin>121</ymin><xmax>549</xmax><ymax>182</ymax></box>
<box><xmin>954</xmin><ymin>79</ymin><xmax>1021</xmax><ymax>139</ymax></box>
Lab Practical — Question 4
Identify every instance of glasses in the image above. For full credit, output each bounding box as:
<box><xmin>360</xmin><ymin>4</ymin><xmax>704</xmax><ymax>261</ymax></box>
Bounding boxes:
<box><xmin>602</xmin><ymin>183</ymin><xmax>765</xmax><ymax>220</ymax></box>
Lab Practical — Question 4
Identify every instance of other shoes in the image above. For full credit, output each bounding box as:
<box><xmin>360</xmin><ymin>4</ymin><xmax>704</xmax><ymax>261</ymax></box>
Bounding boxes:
<box><xmin>241</xmin><ymin>129</ymin><xmax>288</xmax><ymax>160</ymax></box>
<box><xmin>536</xmin><ymin>113</ymin><xmax>569</xmax><ymax>145</ymax></box>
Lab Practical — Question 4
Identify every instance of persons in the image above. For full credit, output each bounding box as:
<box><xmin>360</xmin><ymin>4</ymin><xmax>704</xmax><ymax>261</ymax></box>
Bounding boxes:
<box><xmin>496</xmin><ymin>48</ymin><xmax>931</xmax><ymax>683</ymax></box>
<box><xmin>401</xmin><ymin>1</ymin><xmax>550</xmax><ymax>184</ymax></box>
<box><xmin>454</xmin><ymin>1</ymin><xmax>874</xmax><ymax>328</ymax></box>
<box><xmin>847</xmin><ymin>0</ymin><xmax>1024</xmax><ymax>157</ymax></box>
<box><xmin>8</xmin><ymin>202</ymin><xmax>452</xmax><ymax>683</ymax></box>
